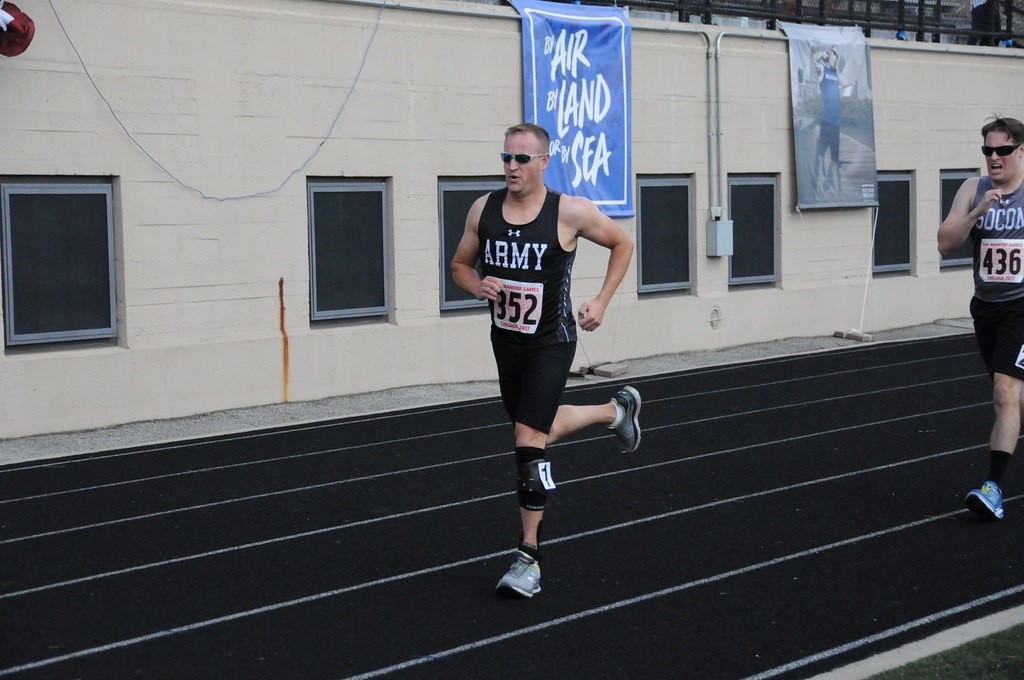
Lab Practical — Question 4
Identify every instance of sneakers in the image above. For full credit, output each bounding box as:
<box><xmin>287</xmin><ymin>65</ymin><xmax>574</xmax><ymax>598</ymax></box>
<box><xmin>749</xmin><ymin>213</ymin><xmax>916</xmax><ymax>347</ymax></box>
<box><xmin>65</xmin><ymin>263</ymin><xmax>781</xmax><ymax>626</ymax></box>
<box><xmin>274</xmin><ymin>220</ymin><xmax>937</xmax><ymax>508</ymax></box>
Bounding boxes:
<box><xmin>964</xmin><ymin>481</ymin><xmax>1004</xmax><ymax>522</ymax></box>
<box><xmin>607</xmin><ymin>386</ymin><xmax>642</xmax><ymax>454</ymax></box>
<box><xmin>495</xmin><ymin>551</ymin><xmax>542</xmax><ymax>602</ymax></box>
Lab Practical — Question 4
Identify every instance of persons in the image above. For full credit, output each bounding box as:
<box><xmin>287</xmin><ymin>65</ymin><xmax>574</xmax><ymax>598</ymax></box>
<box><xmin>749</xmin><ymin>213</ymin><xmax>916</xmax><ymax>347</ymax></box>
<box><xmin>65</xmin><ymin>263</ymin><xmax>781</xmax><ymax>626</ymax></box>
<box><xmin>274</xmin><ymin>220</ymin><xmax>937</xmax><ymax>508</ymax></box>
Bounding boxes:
<box><xmin>806</xmin><ymin>45</ymin><xmax>857</xmax><ymax>204</ymax></box>
<box><xmin>449</xmin><ymin>122</ymin><xmax>642</xmax><ymax>597</ymax></box>
<box><xmin>935</xmin><ymin>115</ymin><xmax>1024</xmax><ymax>519</ymax></box>
<box><xmin>967</xmin><ymin>0</ymin><xmax>1002</xmax><ymax>47</ymax></box>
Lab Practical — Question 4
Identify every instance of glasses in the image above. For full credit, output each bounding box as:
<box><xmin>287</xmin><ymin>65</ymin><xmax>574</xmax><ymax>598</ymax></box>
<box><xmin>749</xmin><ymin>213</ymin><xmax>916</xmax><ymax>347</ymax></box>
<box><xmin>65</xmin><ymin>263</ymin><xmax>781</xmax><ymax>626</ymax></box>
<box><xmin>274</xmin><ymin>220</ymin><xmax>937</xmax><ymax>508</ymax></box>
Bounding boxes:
<box><xmin>500</xmin><ymin>152</ymin><xmax>544</xmax><ymax>164</ymax></box>
<box><xmin>981</xmin><ymin>141</ymin><xmax>1023</xmax><ymax>157</ymax></box>
